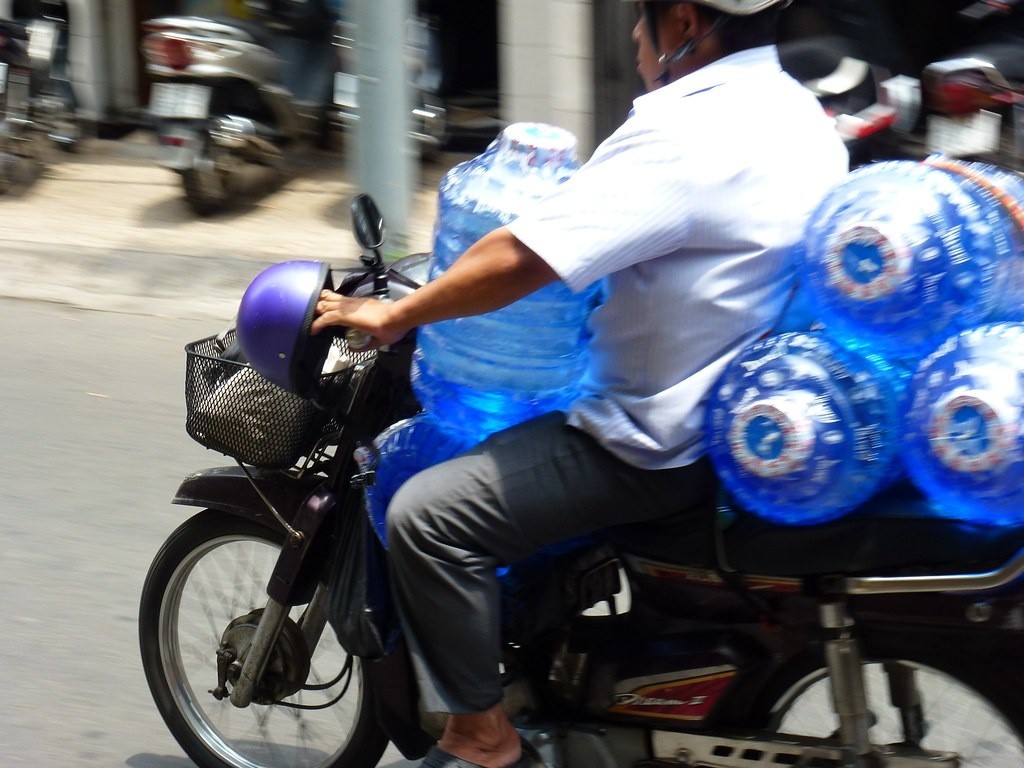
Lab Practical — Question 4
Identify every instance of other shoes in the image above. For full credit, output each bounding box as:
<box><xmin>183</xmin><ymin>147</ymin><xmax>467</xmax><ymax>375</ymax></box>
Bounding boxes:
<box><xmin>420</xmin><ymin>734</ymin><xmax>548</xmax><ymax>768</ymax></box>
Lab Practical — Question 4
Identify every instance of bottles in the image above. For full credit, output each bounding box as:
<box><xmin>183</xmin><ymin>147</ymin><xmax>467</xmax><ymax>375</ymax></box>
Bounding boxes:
<box><xmin>409</xmin><ymin>122</ymin><xmax>608</xmax><ymax>443</ymax></box>
<box><xmin>360</xmin><ymin>408</ymin><xmax>512</xmax><ymax>579</ymax></box>
<box><xmin>788</xmin><ymin>154</ymin><xmax>1024</xmax><ymax>371</ymax></box>
<box><xmin>702</xmin><ymin>329</ymin><xmax>916</xmax><ymax>527</ymax></box>
<box><xmin>890</xmin><ymin>320</ymin><xmax>1024</xmax><ymax>537</ymax></box>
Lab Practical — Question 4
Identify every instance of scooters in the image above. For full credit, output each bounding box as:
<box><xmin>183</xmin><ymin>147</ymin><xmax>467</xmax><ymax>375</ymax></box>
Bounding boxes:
<box><xmin>142</xmin><ymin>2</ymin><xmax>340</xmax><ymax>221</ymax></box>
<box><xmin>329</xmin><ymin>0</ymin><xmax>444</xmax><ymax>169</ymax></box>
<box><xmin>0</xmin><ymin>0</ymin><xmax>83</xmax><ymax>202</ymax></box>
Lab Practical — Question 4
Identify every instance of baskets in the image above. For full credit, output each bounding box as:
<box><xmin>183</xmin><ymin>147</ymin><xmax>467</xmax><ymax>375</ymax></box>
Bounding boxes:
<box><xmin>185</xmin><ymin>328</ymin><xmax>378</xmax><ymax>469</ymax></box>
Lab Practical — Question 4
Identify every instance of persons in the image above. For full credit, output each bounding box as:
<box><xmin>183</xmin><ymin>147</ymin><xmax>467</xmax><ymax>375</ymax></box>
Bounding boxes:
<box><xmin>310</xmin><ymin>0</ymin><xmax>849</xmax><ymax>767</ymax></box>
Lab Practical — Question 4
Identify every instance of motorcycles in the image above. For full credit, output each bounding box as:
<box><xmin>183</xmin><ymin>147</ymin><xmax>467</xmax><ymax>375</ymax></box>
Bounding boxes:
<box><xmin>913</xmin><ymin>0</ymin><xmax>1023</xmax><ymax>170</ymax></box>
<box><xmin>138</xmin><ymin>195</ymin><xmax>1023</xmax><ymax>768</ymax></box>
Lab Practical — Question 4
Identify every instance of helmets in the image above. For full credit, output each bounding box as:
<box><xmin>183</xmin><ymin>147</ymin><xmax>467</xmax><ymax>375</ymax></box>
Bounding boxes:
<box><xmin>697</xmin><ymin>0</ymin><xmax>794</xmax><ymax>16</ymax></box>
<box><xmin>236</xmin><ymin>261</ymin><xmax>334</xmax><ymax>402</ymax></box>
